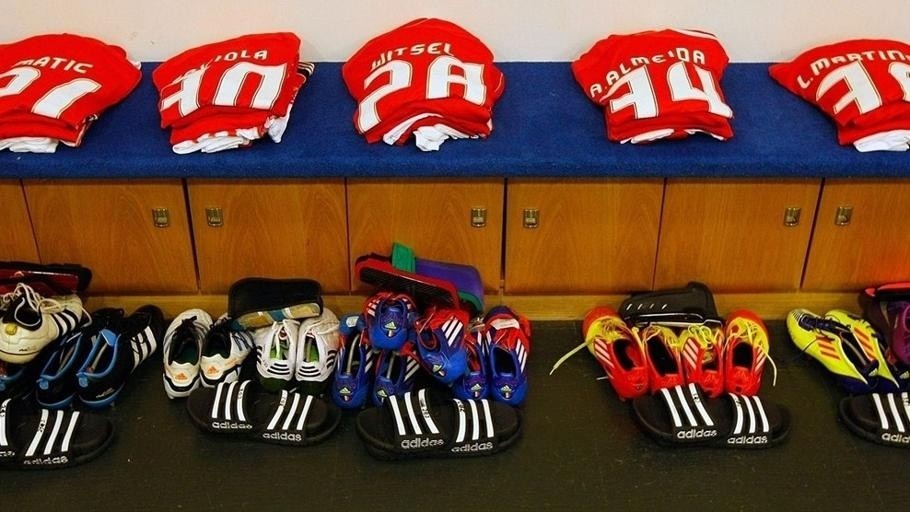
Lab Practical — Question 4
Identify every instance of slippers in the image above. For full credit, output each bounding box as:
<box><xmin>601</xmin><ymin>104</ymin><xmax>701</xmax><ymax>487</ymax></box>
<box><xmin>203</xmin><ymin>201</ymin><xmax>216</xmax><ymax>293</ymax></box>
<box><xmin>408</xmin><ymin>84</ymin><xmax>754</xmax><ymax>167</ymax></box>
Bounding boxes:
<box><xmin>2</xmin><ymin>408</ymin><xmax>114</xmax><ymax>472</ymax></box>
<box><xmin>629</xmin><ymin>382</ymin><xmax>791</xmax><ymax>449</ymax></box>
<box><xmin>841</xmin><ymin>392</ymin><xmax>910</xmax><ymax>447</ymax></box>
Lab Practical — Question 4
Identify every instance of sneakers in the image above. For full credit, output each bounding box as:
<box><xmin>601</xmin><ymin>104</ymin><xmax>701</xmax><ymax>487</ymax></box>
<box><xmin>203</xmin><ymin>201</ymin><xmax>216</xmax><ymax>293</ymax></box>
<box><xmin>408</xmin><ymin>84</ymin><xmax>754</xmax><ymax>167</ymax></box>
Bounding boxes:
<box><xmin>2</xmin><ymin>283</ymin><xmax>163</xmax><ymax>406</ymax></box>
<box><xmin>632</xmin><ymin>321</ymin><xmax>682</xmax><ymax>389</ymax></box>
<box><xmin>679</xmin><ymin>324</ymin><xmax>724</xmax><ymax>395</ymax></box>
<box><xmin>582</xmin><ymin>307</ymin><xmax>648</xmax><ymax>399</ymax></box>
<box><xmin>723</xmin><ymin>309</ymin><xmax>770</xmax><ymax>394</ymax></box>
<box><xmin>787</xmin><ymin>308</ymin><xmax>903</xmax><ymax>392</ymax></box>
